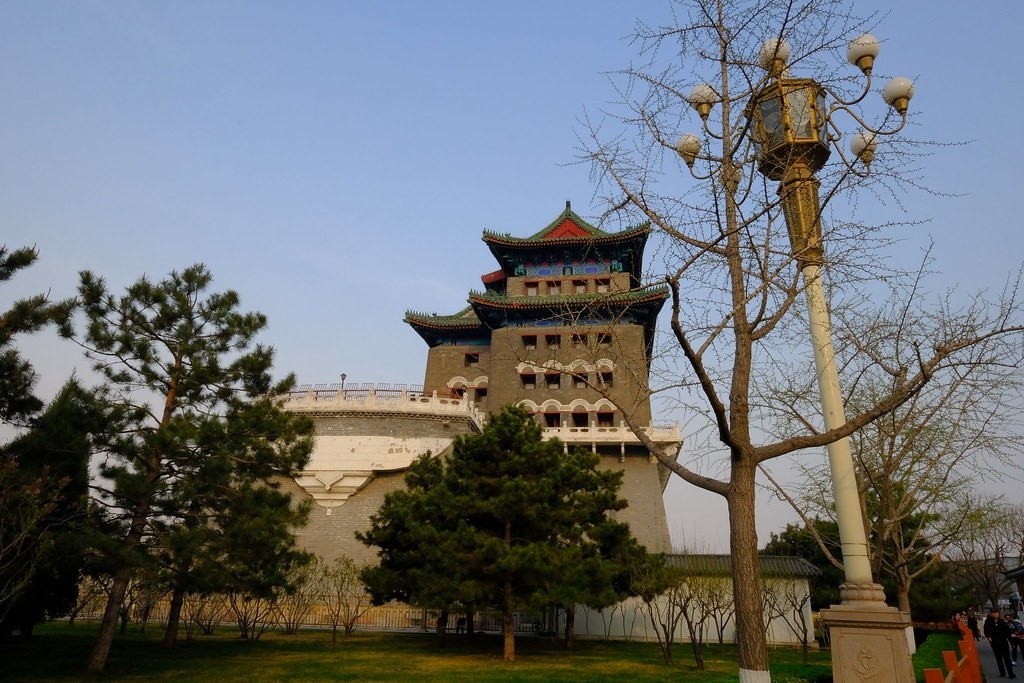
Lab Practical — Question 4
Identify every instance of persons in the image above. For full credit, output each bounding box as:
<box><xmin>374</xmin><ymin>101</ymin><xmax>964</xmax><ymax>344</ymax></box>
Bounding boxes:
<box><xmin>955</xmin><ymin>612</ymin><xmax>1024</xmax><ymax>667</ymax></box>
<box><xmin>984</xmin><ymin>608</ymin><xmax>1016</xmax><ymax>679</ymax></box>
<box><xmin>440</xmin><ymin>609</ymin><xmax>448</xmax><ymax>632</ymax></box>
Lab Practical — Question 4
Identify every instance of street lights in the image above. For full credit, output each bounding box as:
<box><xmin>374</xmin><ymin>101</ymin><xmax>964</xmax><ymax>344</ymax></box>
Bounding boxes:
<box><xmin>676</xmin><ymin>32</ymin><xmax>916</xmax><ymax>683</ymax></box>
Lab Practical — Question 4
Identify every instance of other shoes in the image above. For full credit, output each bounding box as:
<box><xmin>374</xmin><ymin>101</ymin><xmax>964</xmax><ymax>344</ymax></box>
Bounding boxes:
<box><xmin>1012</xmin><ymin>662</ymin><xmax>1016</xmax><ymax>665</ymax></box>
<box><xmin>1009</xmin><ymin>673</ymin><xmax>1016</xmax><ymax>679</ymax></box>
<box><xmin>1000</xmin><ymin>674</ymin><xmax>1005</xmax><ymax>677</ymax></box>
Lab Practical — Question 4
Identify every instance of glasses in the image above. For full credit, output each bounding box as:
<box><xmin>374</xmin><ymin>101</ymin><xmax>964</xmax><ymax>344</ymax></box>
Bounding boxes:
<box><xmin>991</xmin><ymin>611</ymin><xmax>997</xmax><ymax>613</ymax></box>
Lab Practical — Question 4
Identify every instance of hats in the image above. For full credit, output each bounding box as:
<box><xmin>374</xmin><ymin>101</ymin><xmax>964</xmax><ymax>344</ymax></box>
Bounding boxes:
<box><xmin>990</xmin><ymin>608</ymin><xmax>1000</xmax><ymax>613</ymax></box>
